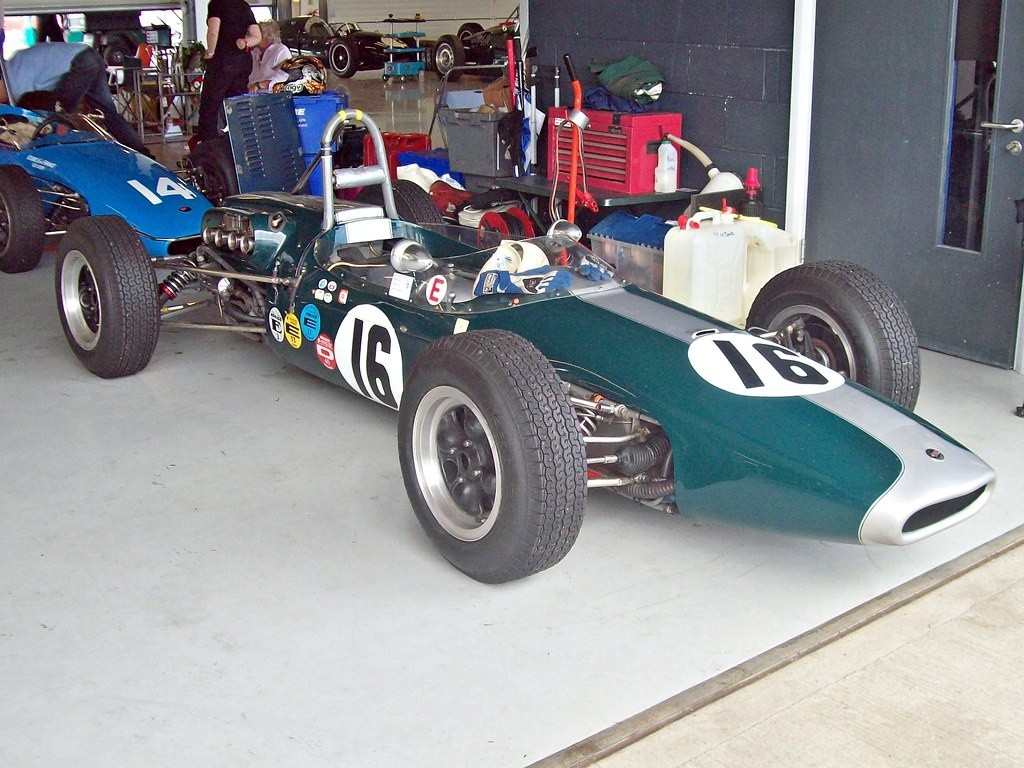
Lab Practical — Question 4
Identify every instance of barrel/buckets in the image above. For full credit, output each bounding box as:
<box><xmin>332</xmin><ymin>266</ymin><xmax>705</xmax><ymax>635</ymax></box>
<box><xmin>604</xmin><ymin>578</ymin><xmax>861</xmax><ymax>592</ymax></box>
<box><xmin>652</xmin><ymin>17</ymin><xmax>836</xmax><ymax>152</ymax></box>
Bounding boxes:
<box><xmin>733</xmin><ymin>212</ymin><xmax>794</xmax><ymax>313</ymax></box>
<box><xmin>662</xmin><ymin>207</ymin><xmax>749</xmax><ymax>327</ymax></box>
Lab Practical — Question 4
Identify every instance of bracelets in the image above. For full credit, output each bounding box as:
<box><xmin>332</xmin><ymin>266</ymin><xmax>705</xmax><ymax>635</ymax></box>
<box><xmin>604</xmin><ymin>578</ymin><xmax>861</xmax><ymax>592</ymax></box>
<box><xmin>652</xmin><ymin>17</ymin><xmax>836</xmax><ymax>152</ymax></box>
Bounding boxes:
<box><xmin>244</xmin><ymin>38</ymin><xmax>249</xmax><ymax>53</ymax></box>
<box><xmin>206</xmin><ymin>50</ymin><xmax>215</xmax><ymax>56</ymax></box>
<box><xmin>254</xmin><ymin>81</ymin><xmax>261</xmax><ymax>90</ymax></box>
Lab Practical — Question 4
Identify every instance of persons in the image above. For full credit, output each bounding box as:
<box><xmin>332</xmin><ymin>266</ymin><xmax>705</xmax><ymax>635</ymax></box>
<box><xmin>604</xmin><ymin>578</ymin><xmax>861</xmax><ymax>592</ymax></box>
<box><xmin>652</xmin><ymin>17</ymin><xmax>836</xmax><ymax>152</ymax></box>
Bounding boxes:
<box><xmin>197</xmin><ymin>0</ymin><xmax>262</xmax><ymax>142</ymax></box>
<box><xmin>35</xmin><ymin>13</ymin><xmax>68</xmax><ymax>45</ymax></box>
<box><xmin>0</xmin><ymin>42</ymin><xmax>160</xmax><ymax>166</ymax></box>
<box><xmin>247</xmin><ymin>20</ymin><xmax>294</xmax><ymax>99</ymax></box>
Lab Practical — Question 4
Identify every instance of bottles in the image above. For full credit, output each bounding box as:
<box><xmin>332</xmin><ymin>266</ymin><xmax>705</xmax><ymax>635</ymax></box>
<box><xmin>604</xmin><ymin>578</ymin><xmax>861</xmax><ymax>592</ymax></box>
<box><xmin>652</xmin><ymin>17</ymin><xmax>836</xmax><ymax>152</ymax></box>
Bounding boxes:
<box><xmin>652</xmin><ymin>138</ymin><xmax>678</xmax><ymax>193</ymax></box>
